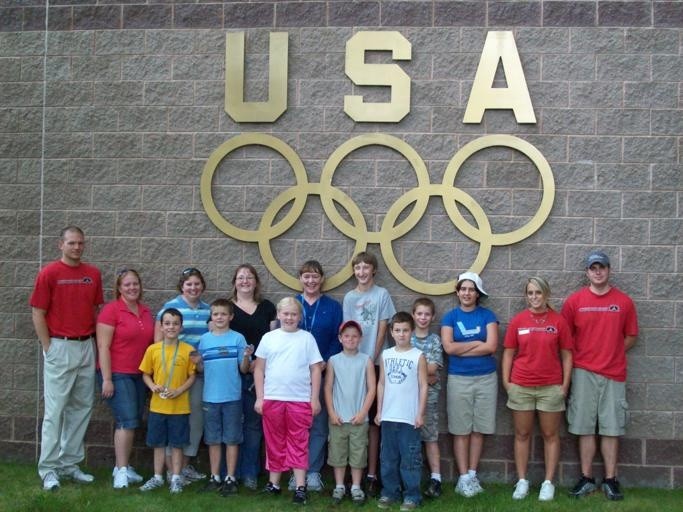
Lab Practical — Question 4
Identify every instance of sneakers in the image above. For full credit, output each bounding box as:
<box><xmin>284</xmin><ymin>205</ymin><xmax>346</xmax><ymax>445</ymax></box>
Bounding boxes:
<box><xmin>537</xmin><ymin>482</ymin><xmax>555</xmax><ymax>501</ymax></box>
<box><xmin>566</xmin><ymin>477</ymin><xmax>597</xmax><ymax>498</ymax></box>
<box><xmin>600</xmin><ymin>476</ymin><xmax>623</xmax><ymax>501</ymax></box>
<box><xmin>287</xmin><ymin>472</ymin><xmax>418</xmax><ymax>511</ymax></box>
<box><xmin>166</xmin><ymin>471</ymin><xmax>192</xmax><ymax>485</ymax></box>
<box><xmin>139</xmin><ymin>477</ymin><xmax>164</xmax><ymax>491</ymax></box>
<box><xmin>62</xmin><ymin>469</ymin><xmax>94</xmax><ymax>483</ymax></box>
<box><xmin>422</xmin><ymin>479</ymin><xmax>442</xmax><ymax>499</ymax></box>
<box><xmin>512</xmin><ymin>479</ymin><xmax>529</xmax><ymax>500</ymax></box>
<box><xmin>198</xmin><ymin>474</ymin><xmax>222</xmax><ymax>495</ymax></box>
<box><xmin>43</xmin><ymin>472</ymin><xmax>60</xmax><ymax>490</ymax></box>
<box><xmin>169</xmin><ymin>477</ymin><xmax>183</xmax><ymax>494</ymax></box>
<box><xmin>113</xmin><ymin>466</ymin><xmax>128</xmax><ymax>489</ymax></box>
<box><xmin>181</xmin><ymin>465</ymin><xmax>207</xmax><ymax>481</ymax></box>
<box><xmin>112</xmin><ymin>465</ymin><xmax>142</xmax><ymax>483</ymax></box>
<box><xmin>219</xmin><ymin>477</ymin><xmax>240</xmax><ymax>497</ymax></box>
<box><xmin>471</xmin><ymin>475</ymin><xmax>484</xmax><ymax>495</ymax></box>
<box><xmin>244</xmin><ymin>476</ymin><xmax>258</xmax><ymax>491</ymax></box>
<box><xmin>259</xmin><ymin>482</ymin><xmax>281</xmax><ymax>495</ymax></box>
<box><xmin>453</xmin><ymin>478</ymin><xmax>477</xmax><ymax>498</ymax></box>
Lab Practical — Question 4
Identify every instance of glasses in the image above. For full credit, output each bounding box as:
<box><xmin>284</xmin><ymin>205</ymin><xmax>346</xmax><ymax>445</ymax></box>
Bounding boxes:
<box><xmin>182</xmin><ymin>267</ymin><xmax>201</xmax><ymax>275</ymax></box>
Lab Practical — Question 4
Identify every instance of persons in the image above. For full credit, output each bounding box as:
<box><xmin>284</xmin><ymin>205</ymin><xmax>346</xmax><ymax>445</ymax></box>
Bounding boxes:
<box><xmin>439</xmin><ymin>271</ymin><xmax>500</xmax><ymax>498</ymax></box>
<box><xmin>153</xmin><ymin>268</ymin><xmax>211</xmax><ymax>487</ymax></box>
<box><xmin>375</xmin><ymin>311</ymin><xmax>429</xmax><ymax>512</ymax></box>
<box><xmin>285</xmin><ymin>259</ymin><xmax>343</xmax><ymax>493</ymax></box>
<box><xmin>407</xmin><ymin>298</ymin><xmax>444</xmax><ymax>499</ymax></box>
<box><xmin>29</xmin><ymin>225</ymin><xmax>104</xmax><ymax>492</ymax></box>
<box><xmin>559</xmin><ymin>251</ymin><xmax>639</xmax><ymax>502</ymax></box>
<box><xmin>342</xmin><ymin>253</ymin><xmax>396</xmax><ymax>498</ymax></box>
<box><xmin>501</xmin><ymin>276</ymin><xmax>573</xmax><ymax>502</ymax></box>
<box><xmin>206</xmin><ymin>262</ymin><xmax>278</xmax><ymax>492</ymax></box>
<box><xmin>138</xmin><ymin>308</ymin><xmax>197</xmax><ymax>495</ymax></box>
<box><xmin>189</xmin><ymin>298</ymin><xmax>256</xmax><ymax>496</ymax></box>
<box><xmin>252</xmin><ymin>297</ymin><xmax>324</xmax><ymax>506</ymax></box>
<box><xmin>95</xmin><ymin>268</ymin><xmax>155</xmax><ymax>490</ymax></box>
<box><xmin>321</xmin><ymin>318</ymin><xmax>377</xmax><ymax>501</ymax></box>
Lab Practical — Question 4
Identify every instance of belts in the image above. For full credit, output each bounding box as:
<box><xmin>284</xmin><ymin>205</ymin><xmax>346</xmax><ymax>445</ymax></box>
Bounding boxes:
<box><xmin>49</xmin><ymin>334</ymin><xmax>96</xmax><ymax>342</ymax></box>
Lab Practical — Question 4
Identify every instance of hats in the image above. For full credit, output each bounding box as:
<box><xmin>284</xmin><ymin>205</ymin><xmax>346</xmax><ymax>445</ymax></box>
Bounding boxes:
<box><xmin>458</xmin><ymin>272</ymin><xmax>489</xmax><ymax>298</ymax></box>
<box><xmin>586</xmin><ymin>250</ymin><xmax>611</xmax><ymax>269</ymax></box>
<box><xmin>339</xmin><ymin>320</ymin><xmax>362</xmax><ymax>334</ymax></box>
